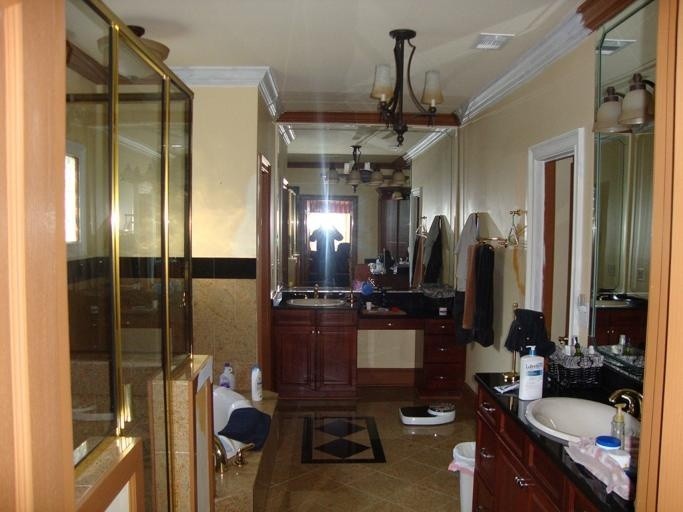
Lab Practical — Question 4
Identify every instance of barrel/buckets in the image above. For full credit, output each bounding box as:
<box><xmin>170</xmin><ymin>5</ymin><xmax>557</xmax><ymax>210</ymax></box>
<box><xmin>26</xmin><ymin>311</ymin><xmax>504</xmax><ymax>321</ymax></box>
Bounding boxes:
<box><xmin>219</xmin><ymin>363</ymin><xmax>236</xmax><ymax>390</ymax></box>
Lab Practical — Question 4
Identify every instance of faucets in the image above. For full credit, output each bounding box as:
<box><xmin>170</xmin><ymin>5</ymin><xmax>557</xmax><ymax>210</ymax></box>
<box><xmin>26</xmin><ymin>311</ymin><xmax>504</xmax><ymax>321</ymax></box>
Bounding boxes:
<box><xmin>609</xmin><ymin>389</ymin><xmax>643</xmax><ymax>414</ymax></box>
<box><xmin>313</xmin><ymin>283</ymin><xmax>320</xmax><ymax>299</ymax></box>
<box><xmin>213</xmin><ymin>434</ymin><xmax>228</xmax><ymax>473</ymax></box>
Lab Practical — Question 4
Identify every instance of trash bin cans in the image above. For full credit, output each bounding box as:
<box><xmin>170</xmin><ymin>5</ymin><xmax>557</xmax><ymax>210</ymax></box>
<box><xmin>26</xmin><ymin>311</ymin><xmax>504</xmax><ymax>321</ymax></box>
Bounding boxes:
<box><xmin>452</xmin><ymin>442</ymin><xmax>476</xmax><ymax>512</ymax></box>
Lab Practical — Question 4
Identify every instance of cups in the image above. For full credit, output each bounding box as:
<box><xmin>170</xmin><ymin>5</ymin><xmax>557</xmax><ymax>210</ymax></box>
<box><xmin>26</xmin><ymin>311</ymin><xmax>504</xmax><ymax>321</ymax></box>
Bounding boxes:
<box><xmin>365</xmin><ymin>303</ymin><xmax>371</xmax><ymax>312</ymax></box>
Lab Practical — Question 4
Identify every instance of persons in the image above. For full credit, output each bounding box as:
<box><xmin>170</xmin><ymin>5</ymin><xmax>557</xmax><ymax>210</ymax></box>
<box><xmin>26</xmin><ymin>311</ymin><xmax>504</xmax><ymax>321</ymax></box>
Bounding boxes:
<box><xmin>311</xmin><ymin>222</ymin><xmax>343</xmax><ymax>280</ymax></box>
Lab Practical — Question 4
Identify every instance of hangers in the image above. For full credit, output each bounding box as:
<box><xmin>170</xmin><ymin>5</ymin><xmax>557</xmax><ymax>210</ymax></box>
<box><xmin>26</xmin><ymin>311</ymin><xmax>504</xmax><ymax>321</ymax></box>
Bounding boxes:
<box><xmin>415</xmin><ymin>216</ymin><xmax>428</xmax><ymax>238</ymax></box>
<box><xmin>507</xmin><ymin>211</ymin><xmax>519</xmax><ymax>247</ymax></box>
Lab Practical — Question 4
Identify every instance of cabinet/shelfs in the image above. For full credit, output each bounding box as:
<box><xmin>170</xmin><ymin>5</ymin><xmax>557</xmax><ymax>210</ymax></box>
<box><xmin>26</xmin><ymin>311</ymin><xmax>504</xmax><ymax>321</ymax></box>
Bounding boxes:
<box><xmin>415</xmin><ymin>321</ymin><xmax>465</xmax><ymax>400</ymax></box>
<box><xmin>377</xmin><ymin>187</ymin><xmax>410</xmax><ymax>265</ymax></box>
<box><xmin>472</xmin><ymin>385</ymin><xmax>599</xmax><ymax>512</ymax></box>
<box><xmin>590</xmin><ymin>308</ymin><xmax>646</xmax><ymax>348</ymax></box>
<box><xmin>272</xmin><ymin>309</ymin><xmax>359</xmax><ymax>402</ymax></box>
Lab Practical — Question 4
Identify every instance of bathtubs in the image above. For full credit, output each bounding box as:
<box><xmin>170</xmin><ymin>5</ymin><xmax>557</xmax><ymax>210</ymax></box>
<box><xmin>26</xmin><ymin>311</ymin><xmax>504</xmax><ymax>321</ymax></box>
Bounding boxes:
<box><xmin>213</xmin><ymin>385</ymin><xmax>254</xmax><ymax>466</ymax></box>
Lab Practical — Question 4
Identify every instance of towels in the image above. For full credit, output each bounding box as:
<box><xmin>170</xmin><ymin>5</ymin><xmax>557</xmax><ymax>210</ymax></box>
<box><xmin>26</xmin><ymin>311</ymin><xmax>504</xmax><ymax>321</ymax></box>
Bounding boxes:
<box><xmin>218</xmin><ymin>408</ymin><xmax>271</xmax><ymax>450</ymax></box>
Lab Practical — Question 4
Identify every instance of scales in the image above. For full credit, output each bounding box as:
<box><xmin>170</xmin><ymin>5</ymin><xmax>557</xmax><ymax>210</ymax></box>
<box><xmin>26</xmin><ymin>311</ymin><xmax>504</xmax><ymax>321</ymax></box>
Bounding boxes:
<box><xmin>399</xmin><ymin>401</ymin><xmax>456</xmax><ymax>425</ymax></box>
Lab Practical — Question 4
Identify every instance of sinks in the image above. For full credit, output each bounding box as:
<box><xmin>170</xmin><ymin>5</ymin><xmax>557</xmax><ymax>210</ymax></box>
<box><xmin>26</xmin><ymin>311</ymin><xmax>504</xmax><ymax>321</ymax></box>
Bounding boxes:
<box><xmin>525</xmin><ymin>396</ymin><xmax>640</xmax><ymax>444</ymax></box>
<box><xmin>286</xmin><ymin>299</ymin><xmax>346</xmax><ymax>307</ymax></box>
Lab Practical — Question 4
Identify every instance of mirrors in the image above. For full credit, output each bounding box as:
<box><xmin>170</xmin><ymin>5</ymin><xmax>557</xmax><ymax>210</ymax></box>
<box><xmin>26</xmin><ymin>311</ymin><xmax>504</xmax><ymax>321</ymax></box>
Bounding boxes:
<box><xmin>589</xmin><ymin>0</ymin><xmax>657</xmax><ymax>383</ymax></box>
<box><xmin>275</xmin><ymin>122</ymin><xmax>458</xmax><ymax>292</ymax></box>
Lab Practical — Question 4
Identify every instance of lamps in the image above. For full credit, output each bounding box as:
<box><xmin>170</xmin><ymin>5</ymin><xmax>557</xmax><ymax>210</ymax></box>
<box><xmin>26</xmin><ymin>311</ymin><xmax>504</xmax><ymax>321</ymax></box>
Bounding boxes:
<box><xmin>391</xmin><ymin>188</ymin><xmax>409</xmax><ymax>201</ymax></box>
<box><xmin>593</xmin><ymin>88</ymin><xmax>626</xmax><ymax>133</ymax></box>
<box><xmin>618</xmin><ymin>73</ymin><xmax>655</xmax><ymax>126</ymax></box>
<box><xmin>370</xmin><ymin>29</ymin><xmax>443</xmax><ymax>146</ymax></box>
<box><xmin>392</xmin><ymin>165</ymin><xmax>409</xmax><ymax>185</ymax></box>
<box><xmin>324</xmin><ymin>145</ymin><xmax>384</xmax><ymax>193</ymax></box>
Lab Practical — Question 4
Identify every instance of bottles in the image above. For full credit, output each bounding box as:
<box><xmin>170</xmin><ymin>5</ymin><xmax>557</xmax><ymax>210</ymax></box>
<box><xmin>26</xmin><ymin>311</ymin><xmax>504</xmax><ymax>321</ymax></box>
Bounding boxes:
<box><xmin>550</xmin><ymin>335</ymin><xmax>603</xmax><ymax>386</ymax></box>
<box><xmin>218</xmin><ymin>362</ymin><xmax>234</xmax><ymax>391</ymax></box>
<box><xmin>250</xmin><ymin>363</ymin><xmax>263</xmax><ymax>402</ymax></box>
<box><xmin>594</xmin><ymin>433</ymin><xmax>637</xmax><ymax>497</ymax></box>
<box><xmin>610</xmin><ymin>335</ymin><xmax>636</xmax><ymax>357</ymax></box>
